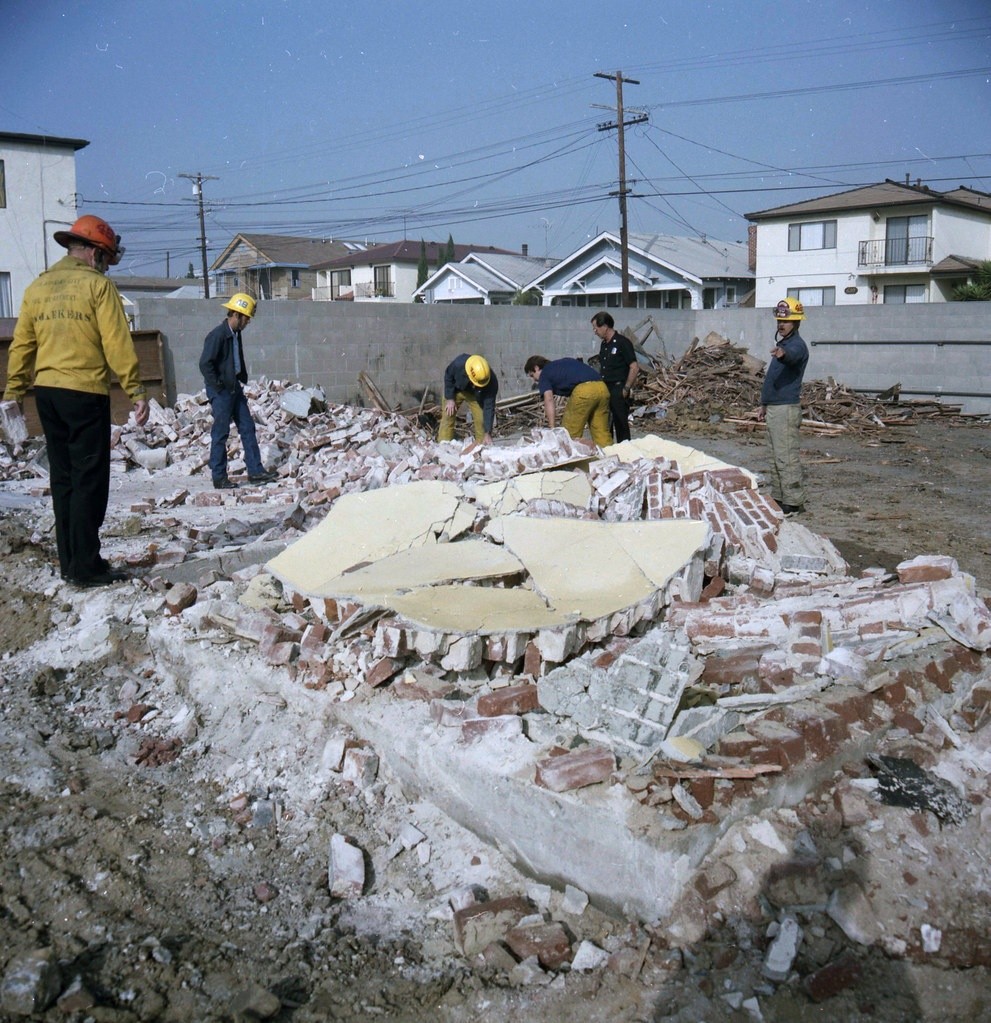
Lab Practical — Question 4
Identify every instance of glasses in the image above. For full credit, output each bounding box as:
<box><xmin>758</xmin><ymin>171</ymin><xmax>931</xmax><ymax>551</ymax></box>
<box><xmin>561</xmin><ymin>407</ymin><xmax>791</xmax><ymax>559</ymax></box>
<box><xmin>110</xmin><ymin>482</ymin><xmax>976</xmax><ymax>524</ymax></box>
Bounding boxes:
<box><xmin>772</xmin><ymin>309</ymin><xmax>788</xmax><ymax>318</ymax></box>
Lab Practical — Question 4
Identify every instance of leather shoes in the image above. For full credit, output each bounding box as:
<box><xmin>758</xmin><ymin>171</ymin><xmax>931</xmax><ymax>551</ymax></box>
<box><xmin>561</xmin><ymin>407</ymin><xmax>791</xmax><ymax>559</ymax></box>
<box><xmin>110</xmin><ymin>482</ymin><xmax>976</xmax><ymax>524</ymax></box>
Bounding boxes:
<box><xmin>61</xmin><ymin>574</ymin><xmax>70</xmax><ymax>579</ymax></box>
<box><xmin>249</xmin><ymin>471</ymin><xmax>279</xmax><ymax>484</ymax></box>
<box><xmin>214</xmin><ymin>479</ymin><xmax>238</xmax><ymax>489</ymax></box>
<box><xmin>68</xmin><ymin>570</ymin><xmax>127</xmax><ymax>586</ymax></box>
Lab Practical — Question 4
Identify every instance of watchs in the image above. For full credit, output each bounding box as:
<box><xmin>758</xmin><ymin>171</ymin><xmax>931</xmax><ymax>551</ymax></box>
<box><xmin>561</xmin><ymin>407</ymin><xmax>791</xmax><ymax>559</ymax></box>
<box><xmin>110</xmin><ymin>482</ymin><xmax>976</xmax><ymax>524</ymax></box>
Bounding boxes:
<box><xmin>624</xmin><ymin>385</ymin><xmax>630</xmax><ymax>393</ymax></box>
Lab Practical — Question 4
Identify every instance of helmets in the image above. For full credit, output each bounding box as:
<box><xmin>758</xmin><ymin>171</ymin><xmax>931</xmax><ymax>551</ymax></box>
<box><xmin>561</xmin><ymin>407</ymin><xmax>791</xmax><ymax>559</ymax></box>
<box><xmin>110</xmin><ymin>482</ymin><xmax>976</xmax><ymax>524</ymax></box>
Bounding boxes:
<box><xmin>222</xmin><ymin>293</ymin><xmax>256</xmax><ymax>317</ymax></box>
<box><xmin>54</xmin><ymin>215</ymin><xmax>117</xmax><ymax>261</ymax></box>
<box><xmin>773</xmin><ymin>298</ymin><xmax>806</xmax><ymax>321</ymax></box>
<box><xmin>465</xmin><ymin>355</ymin><xmax>491</xmax><ymax>387</ymax></box>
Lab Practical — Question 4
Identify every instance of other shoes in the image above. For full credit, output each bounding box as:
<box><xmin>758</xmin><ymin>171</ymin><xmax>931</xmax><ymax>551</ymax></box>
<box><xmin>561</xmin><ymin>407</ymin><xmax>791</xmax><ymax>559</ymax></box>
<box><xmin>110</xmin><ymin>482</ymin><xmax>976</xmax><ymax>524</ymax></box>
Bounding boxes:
<box><xmin>782</xmin><ymin>505</ymin><xmax>805</xmax><ymax>517</ymax></box>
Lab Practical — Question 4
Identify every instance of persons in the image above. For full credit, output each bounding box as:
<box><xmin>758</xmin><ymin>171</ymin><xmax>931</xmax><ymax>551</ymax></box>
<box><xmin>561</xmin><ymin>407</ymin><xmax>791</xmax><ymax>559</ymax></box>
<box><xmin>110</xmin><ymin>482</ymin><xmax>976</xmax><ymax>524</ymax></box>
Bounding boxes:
<box><xmin>435</xmin><ymin>353</ymin><xmax>499</xmax><ymax>447</ymax></box>
<box><xmin>524</xmin><ymin>354</ymin><xmax>611</xmax><ymax>448</ymax></box>
<box><xmin>590</xmin><ymin>312</ymin><xmax>639</xmax><ymax>442</ymax></box>
<box><xmin>199</xmin><ymin>293</ymin><xmax>281</xmax><ymax>489</ymax></box>
<box><xmin>2</xmin><ymin>216</ymin><xmax>150</xmax><ymax>587</ymax></box>
<box><xmin>758</xmin><ymin>297</ymin><xmax>809</xmax><ymax>515</ymax></box>
